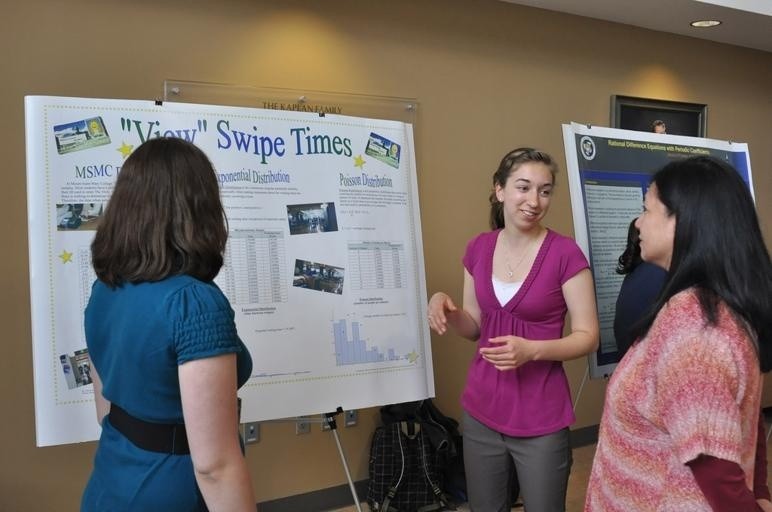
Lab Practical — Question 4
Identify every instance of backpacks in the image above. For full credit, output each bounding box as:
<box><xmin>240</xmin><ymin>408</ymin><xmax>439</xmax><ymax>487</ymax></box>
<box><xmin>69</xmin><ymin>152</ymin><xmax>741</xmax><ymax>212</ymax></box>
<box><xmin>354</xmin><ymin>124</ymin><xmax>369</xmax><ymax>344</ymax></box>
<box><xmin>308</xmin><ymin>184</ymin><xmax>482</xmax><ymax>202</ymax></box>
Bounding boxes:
<box><xmin>366</xmin><ymin>420</ymin><xmax>456</xmax><ymax>510</ymax></box>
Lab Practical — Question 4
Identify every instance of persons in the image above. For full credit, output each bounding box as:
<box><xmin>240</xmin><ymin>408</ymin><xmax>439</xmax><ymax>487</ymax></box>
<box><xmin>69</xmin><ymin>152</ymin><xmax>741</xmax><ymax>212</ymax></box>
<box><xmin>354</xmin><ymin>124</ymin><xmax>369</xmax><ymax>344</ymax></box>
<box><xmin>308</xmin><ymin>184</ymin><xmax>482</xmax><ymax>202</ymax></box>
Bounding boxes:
<box><xmin>425</xmin><ymin>145</ymin><xmax>602</xmax><ymax>511</ymax></box>
<box><xmin>651</xmin><ymin>119</ymin><xmax>666</xmax><ymax>135</ymax></box>
<box><xmin>581</xmin><ymin>154</ymin><xmax>770</xmax><ymax>512</ymax></box>
<box><xmin>78</xmin><ymin>135</ymin><xmax>261</xmax><ymax>511</ymax></box>
<box><xmin>612</xmin><ymin>216</ymin><xmax>677</xmax><ymax>356</ymax></box>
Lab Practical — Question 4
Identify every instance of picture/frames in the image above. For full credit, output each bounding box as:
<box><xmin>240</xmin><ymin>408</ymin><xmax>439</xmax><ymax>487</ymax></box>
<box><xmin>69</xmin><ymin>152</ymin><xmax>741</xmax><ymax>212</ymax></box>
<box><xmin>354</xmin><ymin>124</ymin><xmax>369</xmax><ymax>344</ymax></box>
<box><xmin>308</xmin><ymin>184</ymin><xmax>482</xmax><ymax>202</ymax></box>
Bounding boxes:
<box><xmin>609</xmin><ymin>94</ymin><xmax>708</xmax><ymax>139</ymax></box>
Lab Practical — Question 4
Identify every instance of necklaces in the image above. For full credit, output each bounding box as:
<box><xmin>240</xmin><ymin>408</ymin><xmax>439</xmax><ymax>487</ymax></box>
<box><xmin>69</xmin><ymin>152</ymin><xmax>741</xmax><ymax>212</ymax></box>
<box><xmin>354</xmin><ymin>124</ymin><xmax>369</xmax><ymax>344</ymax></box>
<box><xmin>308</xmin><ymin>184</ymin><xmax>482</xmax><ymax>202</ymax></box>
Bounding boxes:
<box><xmin>500</xmin><ymin>230</ymin><xmax>542</xmax><ymax>280</ymax></box>
